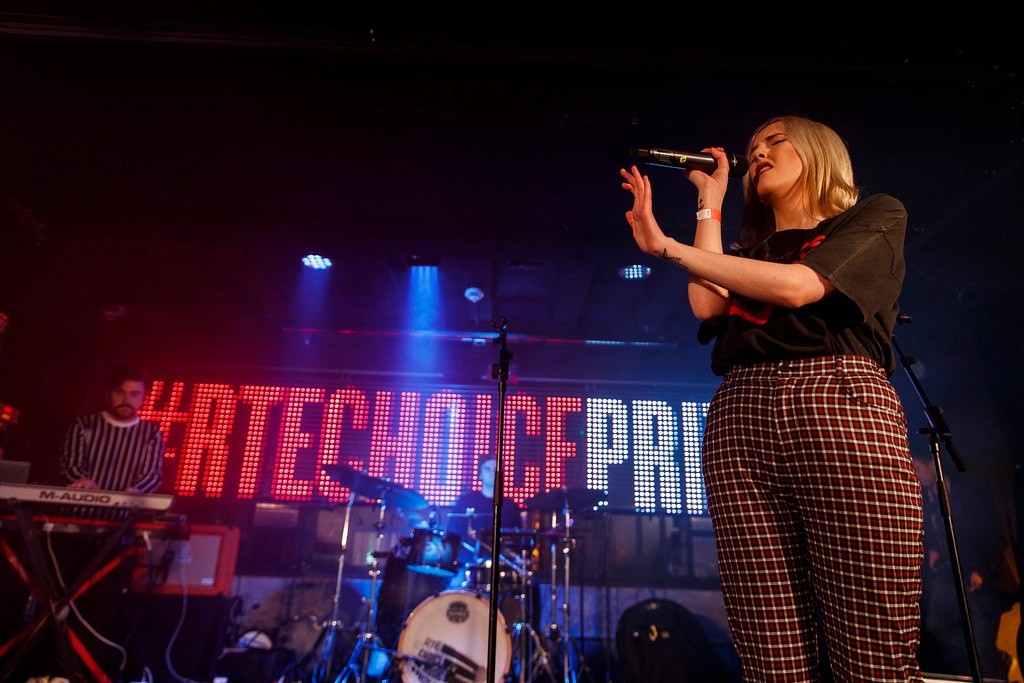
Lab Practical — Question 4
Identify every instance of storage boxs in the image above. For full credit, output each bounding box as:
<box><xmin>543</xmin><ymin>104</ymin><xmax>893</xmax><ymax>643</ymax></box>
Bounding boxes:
<box><xmin>216</xmin><ymin>648</ymin><xmax>294</xmax><ymax>683</ymax></box>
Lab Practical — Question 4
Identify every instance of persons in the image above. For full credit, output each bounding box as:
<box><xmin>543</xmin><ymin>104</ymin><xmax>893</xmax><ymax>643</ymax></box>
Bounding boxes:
<box><xmin>450</xmin><ymin>453</ymin><xmax>522</xmax><ymax>552</ymax></box>
<box><xmin>910</xmin><ymin>448</ymin><xmax>1001</xmax><ymax>661</ymax></box>
<box><xmin>59</xmin><ymin>366</ymin><xmax>165</xmax><ymax>532</ymax></box>
<box><xmin>620</xmin><ymin>116</ymin><xmax>923</xmax><ymax>683</ymax></box>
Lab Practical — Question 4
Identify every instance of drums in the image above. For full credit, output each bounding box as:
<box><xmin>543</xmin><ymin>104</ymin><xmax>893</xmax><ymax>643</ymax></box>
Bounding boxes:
<box><xmin>478</xmin><ymin>526</ymin><xmax>586</xmax><ymax>587</ymax></box>
<box><xmin>372</xmin><ymin>556</ymin><xmax>543</xmax><ymax>683</ymax></box>
<box><xmin>405</xmin><ymin>525</ymin><xmax>461</xmax><ymax>579</ymax></box>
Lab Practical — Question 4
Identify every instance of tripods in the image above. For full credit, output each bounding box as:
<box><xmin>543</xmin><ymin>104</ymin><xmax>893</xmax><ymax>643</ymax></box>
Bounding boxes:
<box><xmin>279</xmin><ymin>488</ymin><xmax>397</xmax><ymax>683</ymax></box>
<box><xmin>505</xmin><ymin>503</ymin><xmax>596</xmax><ymax>683</ymax></box>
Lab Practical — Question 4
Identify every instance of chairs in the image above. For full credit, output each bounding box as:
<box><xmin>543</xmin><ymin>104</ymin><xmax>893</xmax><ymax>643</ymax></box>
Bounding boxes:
<box><xmin>614</xmin><ymin>598</ymin><xmax>732</xmax><ymax>683</ymax></box>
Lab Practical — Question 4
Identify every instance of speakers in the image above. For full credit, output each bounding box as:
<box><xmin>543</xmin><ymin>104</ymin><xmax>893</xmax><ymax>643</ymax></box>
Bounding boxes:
<box><xmin>124</xmin><ymin>522</ymin><xmax>240</xmax><ymax>596</ymax></box>
<box><xmin>113</xmin><ymin>594</ymin><xmax>242</xmax><ymax>683</ymax></box>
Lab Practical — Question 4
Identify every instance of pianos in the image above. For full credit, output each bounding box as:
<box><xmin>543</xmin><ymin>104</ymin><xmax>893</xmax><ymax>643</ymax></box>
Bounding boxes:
<box><xmin>0</xmin><ymin>480</ymin><xmax>191</xmax><ymax>683</ymax></box>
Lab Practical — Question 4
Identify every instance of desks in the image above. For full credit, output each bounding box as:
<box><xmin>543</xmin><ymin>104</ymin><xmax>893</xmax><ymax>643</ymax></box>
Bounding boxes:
<box><xmin>0</xmin><ymin>516</ymin><xmax>191</xmax><ymax>683</ymax></box>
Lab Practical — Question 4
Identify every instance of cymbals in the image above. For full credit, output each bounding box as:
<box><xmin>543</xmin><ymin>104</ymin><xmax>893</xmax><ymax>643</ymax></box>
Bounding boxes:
<box><xmin>521</xmin><ymin>487</ymin><xmax>608</xmax><ymax>510</ymax></box>
<box><xmin>323</xmin><ymin>462</ymin><xmax>428</xmax><ymax>509</ymax></box>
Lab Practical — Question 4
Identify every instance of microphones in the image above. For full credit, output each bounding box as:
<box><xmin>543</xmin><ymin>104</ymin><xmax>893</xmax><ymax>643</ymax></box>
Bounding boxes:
<box><xmin>629</xmin><ymin>146</ymin><xmax>749</xmax><ymax>178</ymax></box>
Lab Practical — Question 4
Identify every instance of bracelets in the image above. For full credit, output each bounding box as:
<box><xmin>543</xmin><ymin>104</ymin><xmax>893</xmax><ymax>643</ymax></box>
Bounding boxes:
<box><xmin>695</xmin><ymin>208</ymin><xmax>721</xmax><ymax>222</ymax></box>
